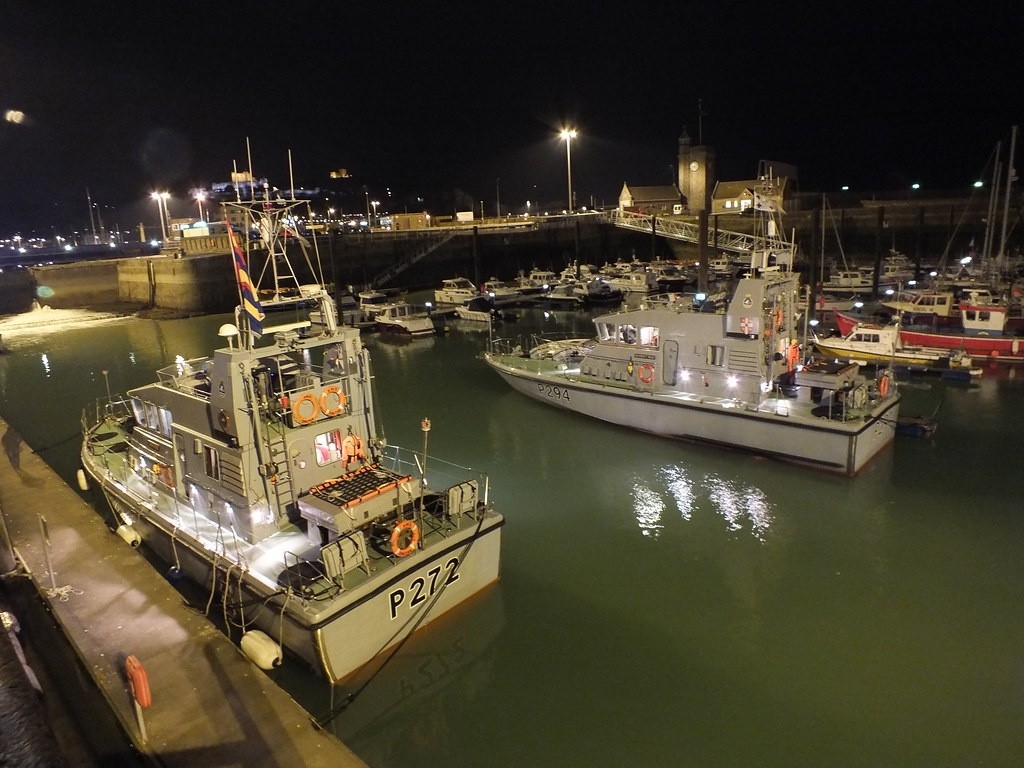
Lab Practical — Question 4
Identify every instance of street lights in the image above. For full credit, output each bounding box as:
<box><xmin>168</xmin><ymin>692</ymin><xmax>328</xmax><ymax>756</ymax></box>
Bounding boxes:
<box><xmin>150</xmin><ymin>192</ymin><xmax>168</xmax><ymax>241</ymax></box>
<box><xmin>327</xmin><ymin>208</ymin><xmax>335</xmax><ymax>220</ymax></box>
<box><xmin>193</xmin><ymin>191</ymin><xmax>205</xmax><ymax>221</ymax></box>
<box><xmin>370</xmin><ymin>201</ymin><xmax>377</xmax><ymax>228</ymax></box>
<box><xmin>0</xmin><ymin>197</ymin><xmax>130</xmax><ymax>250</ymax></box>
<box><xmin>160</xmin><ymin>193</ymin><xmax>171</xmax><ymax>238</ymax></box>
<box><xmin>560</xmin><ymin>130</ymin><xmax>578</xmax><ymax>214</ymax></box>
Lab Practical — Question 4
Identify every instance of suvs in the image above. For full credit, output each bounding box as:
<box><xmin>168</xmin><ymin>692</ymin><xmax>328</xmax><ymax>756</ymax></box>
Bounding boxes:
<box><xmin>339</xmin><ymin>213</ymin><xmax>368</xmax><ymax>232</ymax></box>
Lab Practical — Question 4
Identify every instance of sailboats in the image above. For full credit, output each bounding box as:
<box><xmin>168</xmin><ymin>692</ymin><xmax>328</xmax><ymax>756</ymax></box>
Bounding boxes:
<box><xmin>795</xmin><ymin>124</ymin><xmax>1024</xmax><ymax>380</ymax></box>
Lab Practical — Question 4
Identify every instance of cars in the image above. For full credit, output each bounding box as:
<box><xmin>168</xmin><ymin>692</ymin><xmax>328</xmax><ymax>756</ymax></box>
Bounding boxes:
<box><xmin>672</xmin><ymin>205</ymin><xmax>690</xmax><ymax>216</ymax></box>
<box><xmin>113</xmin><ymin>241</ymin><xmax>161</xmax><ymax>258</ymax></box>
<box><xmin>223</xmin><ymin>228</ymin><xmax>258</xmax><ymax>242</ymax></box>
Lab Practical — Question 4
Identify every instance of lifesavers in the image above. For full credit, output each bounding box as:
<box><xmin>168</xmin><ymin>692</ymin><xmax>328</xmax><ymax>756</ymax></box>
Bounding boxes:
<box><xmin>773</xmin><ymin>307</ymin><xmax>782</xmax><ymax>329</ymax></box>
<box><xmin>879</xmin><ymin>376</ymin><xmax>889</xmax><ymax>399</ymax></box>
<box><xmin>319</xmin><ymin>386</ymin><xmax>346</xmax><ymax>419</ymax></box>
<box><xmin>292</xmin><ymin>394</ymin><xmax>321</xmax><ymax>426</ymax></box>
<box><xmin>390</xmin><ymin>519</ymin><xmax>420</xmax><ymax>557</ymax></box>
<box><xmin>740</xmin><ymin>317</ymin><xmax>754</xmax><ymax>333</ymax></box>
<box><xmin>639</xmin><ymin>363</ymin><xmax>655</xmax><ymax>383</ymax></box>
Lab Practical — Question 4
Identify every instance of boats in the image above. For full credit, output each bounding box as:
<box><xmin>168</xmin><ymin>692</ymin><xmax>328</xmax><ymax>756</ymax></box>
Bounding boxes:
<box><xmin>258</xmin><ymin>256</ymin><xmax>742</xmax><ymax>339</ymax></box>
<box><xmin>483</xmin><ymin>159</ymin><xmax>904</xmax><ymax>480</ymax></box>
<box><xmin>78</xmin><ymin>136</ymin><xmax>506</xmax><ymax>688</ymax></box>
